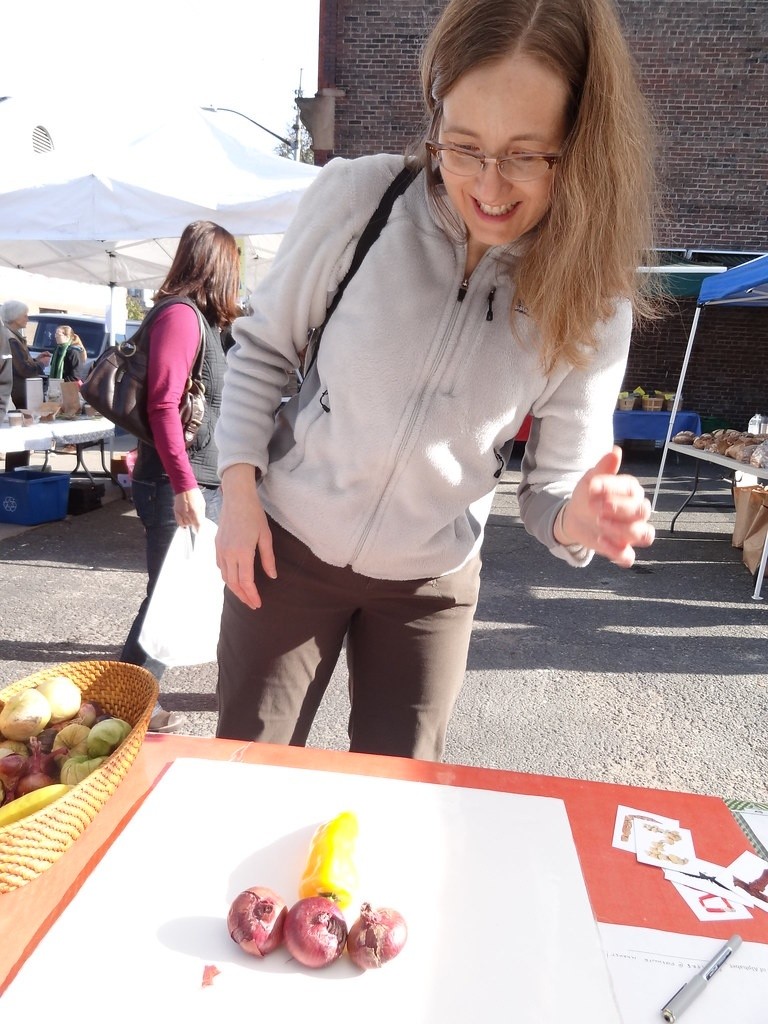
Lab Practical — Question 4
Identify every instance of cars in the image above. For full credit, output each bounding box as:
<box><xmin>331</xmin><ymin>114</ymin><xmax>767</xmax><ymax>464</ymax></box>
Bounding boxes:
<box><xmin>20</xmin><ymin>315</ymin><xmax>142</xmax><ymax>410</ymax></box>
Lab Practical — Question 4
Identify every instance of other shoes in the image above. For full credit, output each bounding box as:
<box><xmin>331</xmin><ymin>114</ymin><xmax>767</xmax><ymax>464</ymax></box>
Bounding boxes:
<box><xmin>147</xmin><ymin>709</ymin><xmax>184</xmax><ymax>733</ymax></box>
<box><xmin>55</xmin><ymin>445</ymin><xmax>77</xmax><ymax>455</ymax></box>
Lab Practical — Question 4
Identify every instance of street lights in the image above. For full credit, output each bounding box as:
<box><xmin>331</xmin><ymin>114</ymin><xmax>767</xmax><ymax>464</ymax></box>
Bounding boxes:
<box><xmin>201</xmin><ymin>66</ymin><xmax>303</xmax><ymax>162</ymax></box>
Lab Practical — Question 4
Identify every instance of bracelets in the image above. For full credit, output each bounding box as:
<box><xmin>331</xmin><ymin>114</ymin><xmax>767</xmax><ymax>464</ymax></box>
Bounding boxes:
<box><xmin>562</xmin><ymin>499</ymin><xmax>579</xmax><ymax>546</ymax></box>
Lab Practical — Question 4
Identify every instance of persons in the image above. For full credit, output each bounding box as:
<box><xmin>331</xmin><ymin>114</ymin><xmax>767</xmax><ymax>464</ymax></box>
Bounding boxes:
<box><xmin>0</xmin><ymin>300</ymin><xmax>88</xmax><ymax>472</ymax></box>
<box><xmin>121</xmin><ymin>219</ymin><xmax>246</xmax><ymax>735</ymax></box>
<box><xmin>213</xmin><ymin>1</ymin><xmax>658</xmax><ymax>766</ymax></box>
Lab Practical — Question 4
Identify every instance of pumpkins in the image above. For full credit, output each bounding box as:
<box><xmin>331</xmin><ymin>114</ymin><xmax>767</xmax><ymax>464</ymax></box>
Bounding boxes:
<box><xmin>0</xmin><ymin>675</ymin><xmax>131</xmax><ymax>755</ymax></box>
<box><xmin>60</xmin><ymin>754</ymin><xmax>109</xmax><ymax>785</ymax></box>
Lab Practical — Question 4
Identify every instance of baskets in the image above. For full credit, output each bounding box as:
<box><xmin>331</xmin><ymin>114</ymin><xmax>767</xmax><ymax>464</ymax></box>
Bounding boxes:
<box><xmin>617</xmin><ymin>394</ymin><xmax>682</xmax><ymax>412</ymax></box>
<box><xmin>0</xmin><ymin>661</ymin><xmax>157</xmax><ymax>897</ymax></box>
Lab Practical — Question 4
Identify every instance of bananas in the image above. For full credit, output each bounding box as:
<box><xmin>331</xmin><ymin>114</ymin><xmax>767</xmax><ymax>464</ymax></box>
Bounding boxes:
<box><xmin>0</xmin><ymin>784</ymin><xmax>70</xmax><ymax>827</ymax></box>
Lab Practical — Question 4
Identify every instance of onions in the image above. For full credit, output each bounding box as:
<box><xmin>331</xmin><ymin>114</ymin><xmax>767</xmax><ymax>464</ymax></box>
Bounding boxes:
<box><xmin>0</xmin><ymin>737</ymin><xmax>70</xmax><ymax>809</ymax></box>
<box><xmin>227</xmin><ymin>885</ymin><xmax>290</xmax><ymax>957</ymax></box>
<box><xmin>281</xmin><ymin>896</ymin><xmax>348</xmax><ymax>967</ymax></box>
<box><xmin>344</xmin><ymin>901</ymin><xmax>408</xmax><ymax>970</ymax></box>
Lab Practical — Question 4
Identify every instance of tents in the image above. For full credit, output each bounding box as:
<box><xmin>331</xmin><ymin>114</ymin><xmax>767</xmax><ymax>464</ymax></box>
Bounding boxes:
<box><xmin>0</xmin><ymin>114</ymin><xmax>322</xmax><ymax>460</ymax></box>
<box><xmin>630</xmin><ymin>254</ymin><xmax>768</xmax><ymax>600</ymax></box>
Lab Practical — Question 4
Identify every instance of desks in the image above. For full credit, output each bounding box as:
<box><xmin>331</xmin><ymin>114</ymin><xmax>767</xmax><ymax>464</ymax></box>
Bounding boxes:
<box><xmin>613</xmin><ymin>408</ymin><xmax>705</xmax><ymax>466</ymax></box>
<box><xmin>0</xmin><ymin>733</ymin><xmax>768</xmax><ymax>1023</ymax></box>
<box><xmin>667</xmin><ymin>442</ymin><xmax>768</xmax><ymax>534</ymax></box>
<box><xmin>0</xmin><ymin>414</ymin><xmax>127</xmax><ymax>508</ymax></box>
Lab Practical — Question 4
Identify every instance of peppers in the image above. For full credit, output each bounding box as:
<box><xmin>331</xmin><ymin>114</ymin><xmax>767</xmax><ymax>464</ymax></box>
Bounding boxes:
<box><xmin>297</xmin><ymin>811</ymin><xmax>360</xmax><ymax>909</ymax></box>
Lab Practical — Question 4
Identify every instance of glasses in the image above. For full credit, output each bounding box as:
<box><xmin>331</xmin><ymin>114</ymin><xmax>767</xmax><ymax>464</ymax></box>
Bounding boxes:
<box><xmin>424</xmin><ymin>102</ymin><xmax>561</xmax><ymax>181</ymax></box>
<box><xmin>55</xmin><ymin>333</ymin><xmax>65</xmax><ymax>338</ymax></box>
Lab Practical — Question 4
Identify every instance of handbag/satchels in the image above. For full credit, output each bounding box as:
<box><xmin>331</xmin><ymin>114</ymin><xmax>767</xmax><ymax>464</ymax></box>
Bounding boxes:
<box><xmin>79</xmin><ymin>295</ymin><xmax>204</xmax><ymax>450</ymax></box>
<box><xmin>732</xmin><ymin>484</ymin><xmax>768</xmax><ymax>576</ymax></box>
<box><xmin>137</xmin><ymin>516</ymin><xmax>225</xmax><ymax>668</ymax></box>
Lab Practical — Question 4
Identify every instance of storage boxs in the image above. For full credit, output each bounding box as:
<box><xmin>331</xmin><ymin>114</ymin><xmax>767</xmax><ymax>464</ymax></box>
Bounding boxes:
<box><xmin>110</xmin><ymin>455</ymin><xmax>129</xmax><ymax>485</ymax></box>
<box><xmin>0</xmin><ymin>470</ymin><xmax>71</xmax><ymax>526</ymax></box>
